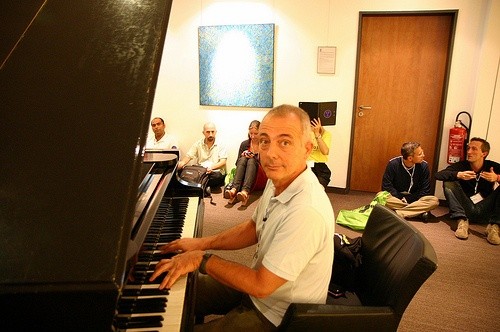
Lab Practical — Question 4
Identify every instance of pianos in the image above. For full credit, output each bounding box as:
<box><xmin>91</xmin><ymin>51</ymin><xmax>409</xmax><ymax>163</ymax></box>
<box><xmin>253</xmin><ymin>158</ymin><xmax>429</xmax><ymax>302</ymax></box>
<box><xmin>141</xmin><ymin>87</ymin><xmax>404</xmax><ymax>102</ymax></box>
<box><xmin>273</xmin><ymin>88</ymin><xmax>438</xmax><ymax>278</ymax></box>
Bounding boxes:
<box><xmin>0</xmin><ymin>0</ymin><xmax>205</xmax><ymax>332</ymax></box>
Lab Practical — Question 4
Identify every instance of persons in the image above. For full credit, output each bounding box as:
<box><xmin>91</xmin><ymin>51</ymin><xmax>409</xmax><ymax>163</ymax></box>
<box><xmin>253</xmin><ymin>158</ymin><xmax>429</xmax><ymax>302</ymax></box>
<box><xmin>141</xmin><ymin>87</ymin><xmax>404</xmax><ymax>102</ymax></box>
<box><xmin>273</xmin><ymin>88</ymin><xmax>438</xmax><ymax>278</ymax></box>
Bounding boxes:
<box><xmin>381</xmin><ymin>142</ymin><xmax>439</xmax><ymax>222</ymax></box>
<box><xmin>434</xmin><ymin>137</ymin><xmax>500</xmax><ymax>245</ymax></box>
<box><xmin>149</xmin><ymin>105</ymin><xmax>334</xmax><ymax>332</ymax></box>
<box><xmin>146</xmin><ymin>118</ymin><xmax>331</xmax><ymax>206</ymax></box>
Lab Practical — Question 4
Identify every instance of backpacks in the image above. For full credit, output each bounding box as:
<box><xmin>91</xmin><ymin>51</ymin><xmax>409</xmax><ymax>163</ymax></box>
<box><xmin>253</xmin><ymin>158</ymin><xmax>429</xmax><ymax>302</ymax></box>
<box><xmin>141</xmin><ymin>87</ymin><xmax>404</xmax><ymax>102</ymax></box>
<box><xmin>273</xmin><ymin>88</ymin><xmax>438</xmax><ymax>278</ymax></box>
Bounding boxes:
<box><xmin>182</xmin><ymin>164</ymin><xmax>216</xmax><ymax>205</ymax></box>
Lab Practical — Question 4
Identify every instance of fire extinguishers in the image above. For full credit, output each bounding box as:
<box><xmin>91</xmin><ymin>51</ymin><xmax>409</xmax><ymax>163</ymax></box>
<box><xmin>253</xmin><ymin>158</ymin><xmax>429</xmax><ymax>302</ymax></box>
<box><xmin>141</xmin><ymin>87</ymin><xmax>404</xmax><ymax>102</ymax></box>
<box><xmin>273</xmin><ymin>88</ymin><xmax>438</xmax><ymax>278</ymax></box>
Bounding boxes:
<box><xmin>446</xmin><ymin>112</ymin><xmax>472</xmax><ymax>164</ymax></box>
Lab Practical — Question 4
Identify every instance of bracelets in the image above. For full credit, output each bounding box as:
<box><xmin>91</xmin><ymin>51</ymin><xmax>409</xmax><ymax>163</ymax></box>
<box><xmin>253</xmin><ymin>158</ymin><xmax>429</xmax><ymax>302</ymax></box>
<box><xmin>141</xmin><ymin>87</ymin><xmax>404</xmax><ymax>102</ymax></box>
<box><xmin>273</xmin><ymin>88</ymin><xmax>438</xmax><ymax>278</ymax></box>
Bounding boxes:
<box><xmin>315</xmin><ymin>135</ymin><xmax>322</xmax><ymax>139</ymax></box>
<box><xmin>198</xmin><ymin>252</ymin><xmax>213</xmax><ymax>274</ymax></box>
<box><xmin>497</xmin><ymin>175</ymin><xmax>499</xmax><ymax>180</ymax></box>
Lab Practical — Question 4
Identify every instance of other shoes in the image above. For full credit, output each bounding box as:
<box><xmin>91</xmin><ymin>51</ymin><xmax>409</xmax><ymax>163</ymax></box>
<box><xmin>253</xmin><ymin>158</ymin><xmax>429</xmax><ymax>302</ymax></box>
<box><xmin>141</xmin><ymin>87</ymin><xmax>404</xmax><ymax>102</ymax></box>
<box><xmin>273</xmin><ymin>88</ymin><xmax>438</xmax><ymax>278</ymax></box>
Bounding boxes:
<box><xmin>455</xmin><ymin>218</ymin><xmax>470</xmax><ymax>239</ymax></box>
<box><xmin>484</xmin><ymin>224</ymin><xmax>500</xmax><ymax>245</ymax></box>
<box><xmin>419</xmin><ymin>212</ymin><xmax>429</xmax><ymax>222</ymax></box>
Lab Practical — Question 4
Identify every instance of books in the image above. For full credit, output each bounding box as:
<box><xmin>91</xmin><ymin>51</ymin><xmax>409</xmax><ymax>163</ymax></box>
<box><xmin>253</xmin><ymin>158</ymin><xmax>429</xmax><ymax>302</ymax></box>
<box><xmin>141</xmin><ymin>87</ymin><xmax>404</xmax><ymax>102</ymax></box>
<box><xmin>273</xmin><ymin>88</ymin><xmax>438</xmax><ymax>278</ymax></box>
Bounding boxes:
<box><xmin>299</xmin><ymin>101</ymin><xmax>337</xmax><ymax>126</ymax></box>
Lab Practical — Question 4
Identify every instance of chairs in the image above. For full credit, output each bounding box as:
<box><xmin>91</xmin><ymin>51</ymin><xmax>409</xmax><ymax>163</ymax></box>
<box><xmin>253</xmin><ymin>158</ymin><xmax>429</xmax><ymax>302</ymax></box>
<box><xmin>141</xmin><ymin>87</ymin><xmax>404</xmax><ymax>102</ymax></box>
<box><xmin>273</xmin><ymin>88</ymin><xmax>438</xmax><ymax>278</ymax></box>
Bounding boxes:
<box><xmin>278</xmin><ymin>204</ymin><xmax>438</xmax><ymax>332</ymax></box>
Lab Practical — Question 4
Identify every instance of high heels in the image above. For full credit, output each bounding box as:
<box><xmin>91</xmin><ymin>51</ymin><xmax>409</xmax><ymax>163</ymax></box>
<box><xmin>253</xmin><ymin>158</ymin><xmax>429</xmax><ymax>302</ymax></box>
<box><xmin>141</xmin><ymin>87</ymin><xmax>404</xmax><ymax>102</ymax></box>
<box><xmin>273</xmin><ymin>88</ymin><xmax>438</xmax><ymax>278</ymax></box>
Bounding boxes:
<box><xmin>225</xmin><ymin>190</ymin><xmax>239</xmax><ymax>204</ymax></box>
<box><xmin>237</xmin><ymin>192</ymin><xmax>249</xmax><ymax>206</ymax></box>
